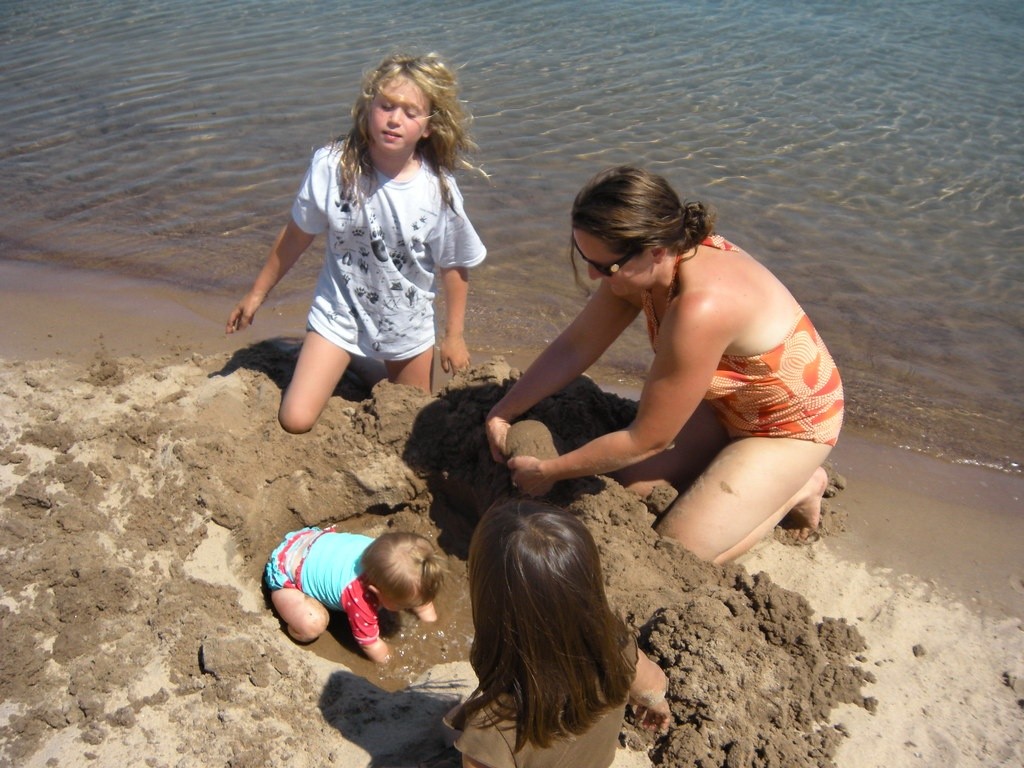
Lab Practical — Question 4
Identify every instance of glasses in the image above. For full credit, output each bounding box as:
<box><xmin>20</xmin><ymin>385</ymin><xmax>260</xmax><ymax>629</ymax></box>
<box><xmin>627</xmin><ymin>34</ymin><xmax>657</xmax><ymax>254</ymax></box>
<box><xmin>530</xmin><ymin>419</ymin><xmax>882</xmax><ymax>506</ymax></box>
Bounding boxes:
<box><xmin>575</xmin><ymin>240</ymin><xmax>637</xmax><ymax>277</ymax></box>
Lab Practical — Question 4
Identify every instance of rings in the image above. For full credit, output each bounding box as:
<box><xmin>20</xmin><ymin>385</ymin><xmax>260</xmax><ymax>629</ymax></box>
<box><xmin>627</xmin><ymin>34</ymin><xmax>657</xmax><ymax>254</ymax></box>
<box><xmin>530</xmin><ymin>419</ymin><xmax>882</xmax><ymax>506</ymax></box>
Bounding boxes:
<box><xmin>513</xmin><ymin>482</ymin><xmax>518</xmax><ymax>488</ymax></box>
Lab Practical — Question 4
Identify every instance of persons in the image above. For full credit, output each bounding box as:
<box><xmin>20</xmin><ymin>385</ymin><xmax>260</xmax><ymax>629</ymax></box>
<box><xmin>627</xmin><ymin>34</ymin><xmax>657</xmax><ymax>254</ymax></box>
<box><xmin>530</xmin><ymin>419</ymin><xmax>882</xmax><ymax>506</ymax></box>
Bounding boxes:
<box><xmin>225</xmin><ymin>49</ymin><xmax>488</xmax><ymax>434</ymax></box>
<box><xmin>485</xmin><ymin>164</ymin><xmax>844</xmax><ymax>564</ymax></box>
<box><xmin>442</xmin><ymin>499</ymin><xmax>671</xmax><ymax>768</ymax></box>
<box><xmin>264</xmin><ymin>527</ymin><xmax>443</xmax><ymax>663</ymax></box>
